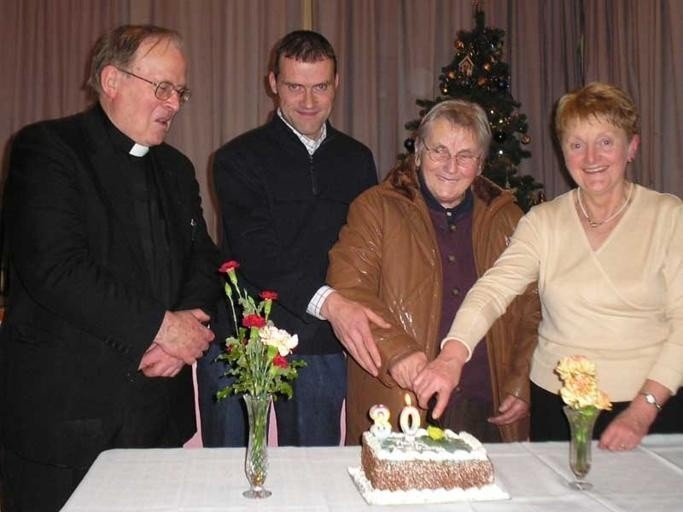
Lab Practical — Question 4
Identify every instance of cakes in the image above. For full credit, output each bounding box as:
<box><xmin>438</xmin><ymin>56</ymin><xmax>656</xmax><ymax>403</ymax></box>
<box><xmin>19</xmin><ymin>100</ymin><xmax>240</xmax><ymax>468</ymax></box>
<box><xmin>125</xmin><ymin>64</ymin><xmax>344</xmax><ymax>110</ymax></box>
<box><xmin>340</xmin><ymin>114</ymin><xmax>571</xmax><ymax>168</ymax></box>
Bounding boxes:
<box><xmin>348</xmin><ymin>399</ymin><xmax>510</xmax><ymax>505</ymax></box>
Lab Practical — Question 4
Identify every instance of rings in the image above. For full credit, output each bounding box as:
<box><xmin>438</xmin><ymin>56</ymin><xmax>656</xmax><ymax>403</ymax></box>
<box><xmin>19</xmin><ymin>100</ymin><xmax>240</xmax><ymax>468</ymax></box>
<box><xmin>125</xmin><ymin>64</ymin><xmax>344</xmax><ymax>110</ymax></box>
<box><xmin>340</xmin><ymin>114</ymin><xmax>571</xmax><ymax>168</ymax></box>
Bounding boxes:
<box><xmin>620</xmin><ymin>444</ymin><xmax>626</xmax><ymax>449</ymax></box>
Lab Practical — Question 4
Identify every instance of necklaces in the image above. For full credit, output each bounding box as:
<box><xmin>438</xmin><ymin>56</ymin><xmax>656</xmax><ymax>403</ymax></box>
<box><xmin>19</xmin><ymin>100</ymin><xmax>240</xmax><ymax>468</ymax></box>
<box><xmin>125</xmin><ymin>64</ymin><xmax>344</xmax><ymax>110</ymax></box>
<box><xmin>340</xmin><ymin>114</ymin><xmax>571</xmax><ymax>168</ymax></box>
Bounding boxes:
<box><xmin>577</xmin><ymin>182</ymin><xmax>634</xmax><ymax>229</ymax></box>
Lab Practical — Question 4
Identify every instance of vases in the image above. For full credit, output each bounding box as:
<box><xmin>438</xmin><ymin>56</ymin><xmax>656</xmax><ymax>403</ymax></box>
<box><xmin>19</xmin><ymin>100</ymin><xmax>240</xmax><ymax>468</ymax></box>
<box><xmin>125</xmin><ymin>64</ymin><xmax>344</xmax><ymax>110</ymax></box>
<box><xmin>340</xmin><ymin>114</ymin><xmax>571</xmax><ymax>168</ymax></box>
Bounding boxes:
<box><xmin>243</xmin><ymin>394</ymin><xmax>273</xmax><ymax>499</ymax></box>
<box><xmin>562</xmin><ymin>405</ymin><xmax>601</xmax><ymax>492</ymax></box>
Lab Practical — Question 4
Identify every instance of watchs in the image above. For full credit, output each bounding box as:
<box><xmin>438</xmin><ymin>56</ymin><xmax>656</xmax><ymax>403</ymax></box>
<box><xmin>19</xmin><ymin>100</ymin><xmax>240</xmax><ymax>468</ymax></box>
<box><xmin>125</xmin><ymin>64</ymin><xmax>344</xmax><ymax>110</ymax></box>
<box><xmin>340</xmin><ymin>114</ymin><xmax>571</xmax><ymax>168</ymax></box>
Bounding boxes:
<box><xmin>637</xmin><ymin>390</ymin><xmax>663</xmax><ymax>412</ymax></box>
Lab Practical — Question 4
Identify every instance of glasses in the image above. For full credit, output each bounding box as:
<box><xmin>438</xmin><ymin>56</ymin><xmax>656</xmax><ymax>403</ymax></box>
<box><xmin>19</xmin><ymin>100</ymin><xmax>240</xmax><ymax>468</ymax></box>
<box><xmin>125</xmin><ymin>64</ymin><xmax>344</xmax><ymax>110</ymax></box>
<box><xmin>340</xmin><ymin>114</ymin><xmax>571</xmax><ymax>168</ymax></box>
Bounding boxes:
<box><xmin>419</xmin><ymin>136</ymin><xmax>481</xmax><ymax>168</ymax></box>
<box><xmin>116</xmin><ymin>65</ymin><xmax>191</xmax><ymax>105</ymax></box>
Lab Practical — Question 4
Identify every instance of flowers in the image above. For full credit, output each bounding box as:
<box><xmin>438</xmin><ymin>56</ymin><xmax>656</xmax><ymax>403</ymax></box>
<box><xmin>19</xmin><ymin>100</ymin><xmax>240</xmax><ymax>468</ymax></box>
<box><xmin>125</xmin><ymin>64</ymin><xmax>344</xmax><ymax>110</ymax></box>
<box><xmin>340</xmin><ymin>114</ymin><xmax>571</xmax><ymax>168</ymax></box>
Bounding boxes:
<box><xmin>554</xmin><ymin>355</ymin><xmax>613</xmax><ymax>472</ymax></box>
<box><xmin>211</xmin><ymin>259</ymin><xmax>305</xmax><ymax>486</ymax></box>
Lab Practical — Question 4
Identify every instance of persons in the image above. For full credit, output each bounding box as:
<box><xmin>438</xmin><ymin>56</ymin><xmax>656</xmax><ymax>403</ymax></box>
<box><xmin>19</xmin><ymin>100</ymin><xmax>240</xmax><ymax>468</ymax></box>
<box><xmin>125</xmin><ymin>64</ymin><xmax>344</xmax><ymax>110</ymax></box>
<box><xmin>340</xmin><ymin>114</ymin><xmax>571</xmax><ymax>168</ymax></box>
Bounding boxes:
<box><xmin>323</xmin><ymin>100</ymin><xmax>542</xmax><ymax>444</ymax></box>
<box><xmin>412</xmin><ymin>80</ymin><xmax>683</xmax><ymax>450</ymax></box>
<box><xmin>0</xmin><ymin>21</ymin><xmax>225</xmax><ymax>512</ymax></box>
<box><xmin>205</xmin><ymin>26</ymin><xmax>378</xmax><ymax>445</ymax></box>
